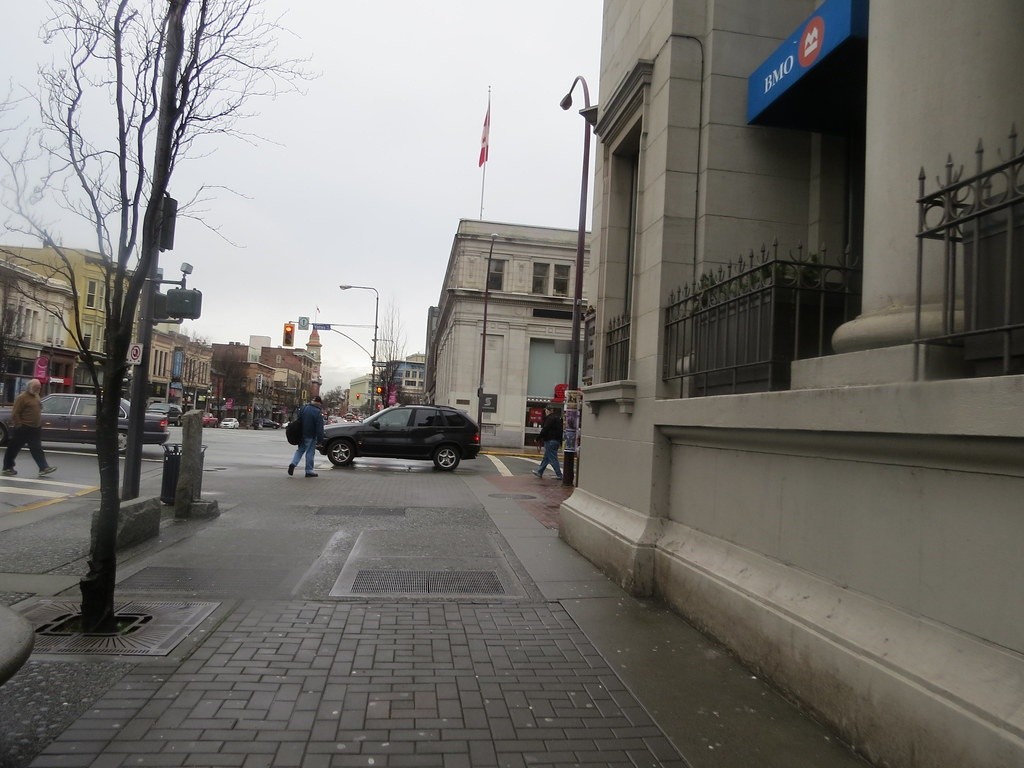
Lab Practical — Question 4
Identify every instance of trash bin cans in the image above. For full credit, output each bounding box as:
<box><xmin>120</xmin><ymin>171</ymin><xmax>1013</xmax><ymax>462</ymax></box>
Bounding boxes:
<box><xmin>159</xmin><ymin>443</ymin><xmax>208</xmax><ymax>506</ymax></box>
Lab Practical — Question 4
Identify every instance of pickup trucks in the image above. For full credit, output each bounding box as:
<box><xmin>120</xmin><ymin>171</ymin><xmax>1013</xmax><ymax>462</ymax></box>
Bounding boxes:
<box><xmin>202</xmin><ymin>413</ymin><xmax>218</xmax><ymax>428</ymax></box>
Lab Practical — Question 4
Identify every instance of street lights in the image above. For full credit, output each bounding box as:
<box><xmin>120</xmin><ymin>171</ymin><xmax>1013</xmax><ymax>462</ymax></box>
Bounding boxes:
<box><xmin>262</xmin><ymin>371</ymin><xmax>287</xmax><ymax>416</ymax></box>
<box><xmin>340</xmin><ymin>286</ymin><xmax>378</xmax><ymax>417</ymax></box>
<box><xmin>478</xmin><ymin>234</ymin><xmax>499</xmax><ymax>449</ymax></box>
<box><xmin>561</xmin><ymin>76</ymin><xmax>590</xmax><ymax>487</ymax></box>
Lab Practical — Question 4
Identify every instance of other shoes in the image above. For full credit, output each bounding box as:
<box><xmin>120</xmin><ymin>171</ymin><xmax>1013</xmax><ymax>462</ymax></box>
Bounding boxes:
<box><xmin>288</xmin><ymin>463</ymin><xmax>295</xmax><ymax>475</ymax></box>
<box><xmin>531</xmin><ymin>470</ymin><xmax>542</xmax><ymax>478</ymax></box>
<box><xmin>305</xmin><ymin>472</ymin><xmax>318</xmax><ymax>477</ymax></box>
<box><xmin>0</xmin><ymin>469</ymin><xmax>17</xmax><ymax>476</ymax></box>
<box><xmin>38</xmin><ymin>467</ymin><xmax>57</xmax><ymax>477</ymax></box>
<box><xmin>556</xmin><ymin>476</ymin><xmax>563</xmax><ymax>480</ymax></box>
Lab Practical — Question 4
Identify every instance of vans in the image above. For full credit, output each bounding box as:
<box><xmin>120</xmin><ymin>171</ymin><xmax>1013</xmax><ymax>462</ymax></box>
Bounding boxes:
<box><xmin>359</xmin><ymin>417</ymin><xmax>364</xmax><ymax>422</ymax></box>
<box><xmin>346</xmin><ymin>414</ymin><xmax>352</xmax><ymax>420</ymax></box>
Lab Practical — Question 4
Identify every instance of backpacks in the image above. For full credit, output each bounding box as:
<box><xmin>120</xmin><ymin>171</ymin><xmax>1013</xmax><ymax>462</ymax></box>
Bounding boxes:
<box><xmin>286</xmin><ymin>404</ymin><xmax>307</xmax><ymax>446</ymax></box>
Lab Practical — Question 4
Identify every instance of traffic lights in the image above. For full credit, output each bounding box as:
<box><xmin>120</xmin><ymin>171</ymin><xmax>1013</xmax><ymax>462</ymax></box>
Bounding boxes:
<box><xmin>283</xmin><ymin>324</ymin><xmax>295</xmax><ymax>346</ymax></box>
<box><xmin>376</xmin><ymin>386</ymin><xmax>384</xmax><ymax>394</ymax></box>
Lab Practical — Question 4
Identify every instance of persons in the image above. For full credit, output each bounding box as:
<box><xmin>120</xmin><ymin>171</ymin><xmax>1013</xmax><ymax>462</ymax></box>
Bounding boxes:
<box><xmin>0</xmin><ymin>379</ymin><xmax>57</xmax><ymax>476</ymax></box>
<box><xmin>288</xmin><ymin>396</ymin><xmax>324</xmax><ymax>477</ymax></box>
<box><xmin>532</xmin><ymin>404</ymin><xmax>563</xmax><ymax>479</ymax></box>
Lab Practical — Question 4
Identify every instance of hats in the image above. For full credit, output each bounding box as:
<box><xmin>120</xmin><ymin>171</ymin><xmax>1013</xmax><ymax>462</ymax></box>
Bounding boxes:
<box><xmin>312</xmin><ymin>396</ymin><xmax>323</xmax><ymax>404</ymax></box>
<box><xmin>543</xmin><ymin>404</ymin><xmax>554</xmax><ymax>409</ymax></box>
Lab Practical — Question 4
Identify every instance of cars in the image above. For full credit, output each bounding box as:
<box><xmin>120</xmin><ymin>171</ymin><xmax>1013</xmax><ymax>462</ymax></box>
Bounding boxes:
<box><xmin>329</xmin><ymin>416</ymin><xmax>336</xmax><ymax>422</ymax></box>
<box><xmin>220</xmin><ymin>418</ymin><xmax>239</xmax><ymax>429</ymax></box>
<box><xmin>0</xmin><ymin>393</ymin><xmax>170</xmax><ymax>455</ymax></box>
<box><xmin>281</xmin><ymin>421</ymin><xmax>292</xmax><ymax>428</ymax></box>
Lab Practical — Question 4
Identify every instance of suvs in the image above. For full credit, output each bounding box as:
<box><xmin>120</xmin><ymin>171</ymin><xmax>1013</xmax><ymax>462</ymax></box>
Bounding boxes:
<box><xmin>146</xmin><ymin>403</ymin><xmax>182</xmax><ymax>427</ymax></box>
<box><xmin>315</xmin><ymin>403</ymin><xmax>481</xmax><ymax>472</ymax></box>
<box><xmin>252</xmin><ymin>419</ymin><xmax>279</xmax><ymax>429</ymax></box>
